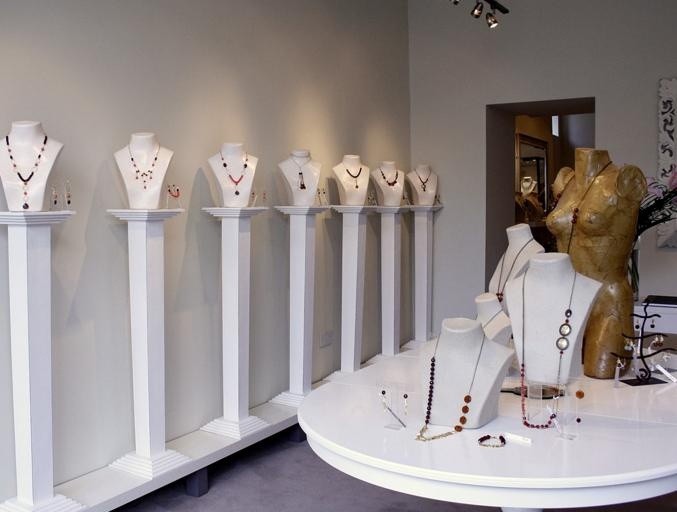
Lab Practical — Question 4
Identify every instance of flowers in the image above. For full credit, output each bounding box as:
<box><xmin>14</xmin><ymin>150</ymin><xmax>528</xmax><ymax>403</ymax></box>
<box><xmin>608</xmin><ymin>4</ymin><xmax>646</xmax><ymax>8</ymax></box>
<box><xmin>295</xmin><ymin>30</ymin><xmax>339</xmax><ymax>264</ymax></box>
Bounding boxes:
<box><xmin>622</xmin><ymin>162</ymin><xmax>677</xmax><ymax>294</ymax></box>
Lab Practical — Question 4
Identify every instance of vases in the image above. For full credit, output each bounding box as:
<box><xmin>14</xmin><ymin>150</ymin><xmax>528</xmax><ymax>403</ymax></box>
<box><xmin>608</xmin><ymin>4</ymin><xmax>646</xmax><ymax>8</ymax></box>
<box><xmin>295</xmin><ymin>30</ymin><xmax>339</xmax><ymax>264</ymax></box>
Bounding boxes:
<box><xmin>627</xmin><ymin>240</ymin><xmax>641</xmax><ymax>302</ymax></box>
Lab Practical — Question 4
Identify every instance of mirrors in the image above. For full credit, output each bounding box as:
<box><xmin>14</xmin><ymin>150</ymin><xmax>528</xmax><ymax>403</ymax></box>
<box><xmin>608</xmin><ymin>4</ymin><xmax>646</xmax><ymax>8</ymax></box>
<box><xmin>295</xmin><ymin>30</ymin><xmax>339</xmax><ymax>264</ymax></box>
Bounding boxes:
<box><xmin>516</xmin><ymin>133</ymin><xmax>549</xmax><ymax>215</ymax></box>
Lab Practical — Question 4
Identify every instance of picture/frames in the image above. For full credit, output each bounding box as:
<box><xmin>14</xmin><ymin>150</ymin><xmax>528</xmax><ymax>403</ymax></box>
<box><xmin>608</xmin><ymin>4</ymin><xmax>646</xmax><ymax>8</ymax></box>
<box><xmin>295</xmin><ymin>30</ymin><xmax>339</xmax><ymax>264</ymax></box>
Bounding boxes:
<box><xmin>656</xmin><ymin>75</ymin><xmax>677</xmax><ymax>250</ymax></box>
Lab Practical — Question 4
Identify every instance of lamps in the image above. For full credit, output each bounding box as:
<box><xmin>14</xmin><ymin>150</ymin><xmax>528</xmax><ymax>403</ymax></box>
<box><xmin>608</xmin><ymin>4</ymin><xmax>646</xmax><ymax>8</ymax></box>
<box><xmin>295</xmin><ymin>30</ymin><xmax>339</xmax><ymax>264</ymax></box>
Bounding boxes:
<box><xmin>450</xmin><ymin>0</ymin><xmax>509</xmax><ymax>28</ymax></box>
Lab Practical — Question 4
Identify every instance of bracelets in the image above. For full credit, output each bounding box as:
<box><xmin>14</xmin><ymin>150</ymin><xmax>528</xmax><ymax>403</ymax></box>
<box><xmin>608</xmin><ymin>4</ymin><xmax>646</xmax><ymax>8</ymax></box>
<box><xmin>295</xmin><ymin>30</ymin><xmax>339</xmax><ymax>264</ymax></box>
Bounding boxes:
<box><xmin>166</xmin><ymin>183</ymin><xmax>180</xmax><ymax>198</ymax></box>
<box><xmin>477</xmin><ymin>434</ymin><xmax>506</xmax><ymax>447</ymax></box>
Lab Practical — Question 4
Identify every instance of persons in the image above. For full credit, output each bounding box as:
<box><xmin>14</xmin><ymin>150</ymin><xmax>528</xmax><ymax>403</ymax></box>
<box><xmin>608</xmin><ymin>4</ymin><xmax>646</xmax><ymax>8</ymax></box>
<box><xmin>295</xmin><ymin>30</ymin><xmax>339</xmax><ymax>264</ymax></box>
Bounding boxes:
<box><xmin>545</xmin><ymin>145</ymin><xmax>649</xmax><ymax>380</ymax></box>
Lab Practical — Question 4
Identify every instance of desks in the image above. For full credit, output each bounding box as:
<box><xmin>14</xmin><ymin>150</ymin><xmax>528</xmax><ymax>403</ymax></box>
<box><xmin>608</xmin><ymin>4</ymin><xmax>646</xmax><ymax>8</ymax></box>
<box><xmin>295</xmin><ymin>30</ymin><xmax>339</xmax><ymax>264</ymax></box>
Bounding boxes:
<box><xmin>296</xmin><ymin>339</ymin><xmax>677</xmax><ymax>512</ymax></box>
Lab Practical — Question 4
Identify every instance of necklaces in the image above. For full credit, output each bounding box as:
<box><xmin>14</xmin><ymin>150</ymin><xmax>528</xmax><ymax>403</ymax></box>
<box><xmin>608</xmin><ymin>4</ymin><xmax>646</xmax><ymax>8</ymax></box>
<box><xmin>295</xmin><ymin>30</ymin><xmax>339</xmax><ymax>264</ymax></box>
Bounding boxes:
<box><xmin>288</xmin><ymin>153</ymin><xmax>313</xmax><ymax>192</ymax></box>
<box><xmin>473</xmin><ymin>307</ymin><xmax>504</xmax><ymax>328</ymax></box>
<box><xmin>378</xmin><ymin>166</ymin><xmax>399</xmax><ymax>187</ymax></box>
<box><xmin>414</xmin><ymin>169</ymin><xmax>432</xmax><ymax>192</ymax></box>
<box><xmin>413</xmin><ymin>329</ymin><xmax>486</xmax><ymax>441</ymax></box>
<box><xmin>547</xmin><ymin>160</ymin><xmax>612</xmax><ymax>254</ymax></box>
<box><xmin>339</xmin><ymin>160</ymin><xmax>365</xmax><ymax>190</ymax></box>
<box><xmin>519</xmin><ymin>265</ymin><xmax>577</xmax><ymax>429</ymax></box>
<box><xmin>493</xmin><ymin>237</ymin><xmax>535</xmax><ymax>304</ymax></box>
<box><xmin>125</xmin><ymin>140</ymin><xmax>161</xmax><ymax>192</ymax></box>
<box><xmin>5</xmin><ymin>131</ymin><xmax>49</xmax><ymax>211</ymax></box>
<box><xmin>520</xmin><ymin>179</ymin><xmax>533</xmax><ymax>193</ymax></box>
<box><xmin>217</xmin><ymin>148</ymin><xmax>249</xmax><ymax>197</ymax></box>
